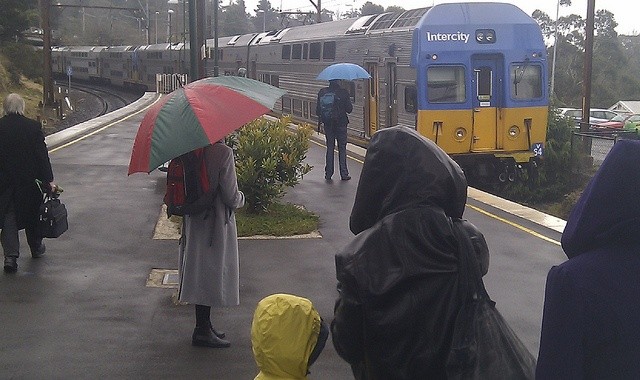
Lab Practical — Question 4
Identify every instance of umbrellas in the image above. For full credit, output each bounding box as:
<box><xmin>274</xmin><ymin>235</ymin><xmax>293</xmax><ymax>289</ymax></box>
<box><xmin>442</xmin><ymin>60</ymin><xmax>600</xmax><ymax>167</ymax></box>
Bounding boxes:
<box><xmin>315</xmin><ymin>63</ymin><xmax>373</xmax><ymax>92</ymax></box>
<box><xmin>127</xmin><ymin>74</ymin><xmax>290</xmax><ymax>176</ymax></box>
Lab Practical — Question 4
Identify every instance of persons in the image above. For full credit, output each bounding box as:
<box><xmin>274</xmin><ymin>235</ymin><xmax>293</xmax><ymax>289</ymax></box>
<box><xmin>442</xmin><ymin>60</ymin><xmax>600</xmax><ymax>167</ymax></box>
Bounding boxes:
<box><xmin>535</xmin><ymin>139</ymin><xmax>640</xmax><ymax>380</ymax></box>
<box><xmin>330</xmin><ymin>125</ymin><xmax>538</xmax><ymax>379</ymax></box>
<box><xmin>177</xmin><ymin>136</ymin><xmax>244</xmax><ymax>347</ymax></box>
<box><xmin>0</xmin><ymin>92</ymin><xmax>54</xmax><ymax>272</ymax></box>
<box><xmin>316</xmin><ymin>80</ymin><xmax>353</xmax><ymax>180</ymax></box>
<box><xmin>250</xmin><ymin>294</ymin><xmax>329</xmax><ymax>380</ymax></box>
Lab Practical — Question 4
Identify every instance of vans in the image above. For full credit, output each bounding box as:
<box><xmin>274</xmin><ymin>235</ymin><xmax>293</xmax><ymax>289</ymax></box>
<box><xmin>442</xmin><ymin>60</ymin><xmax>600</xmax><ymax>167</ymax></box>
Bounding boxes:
<box><xmin>555</xmin><ymin>108</ymin><xmax>625</xmax><ymax>126</ymax></box>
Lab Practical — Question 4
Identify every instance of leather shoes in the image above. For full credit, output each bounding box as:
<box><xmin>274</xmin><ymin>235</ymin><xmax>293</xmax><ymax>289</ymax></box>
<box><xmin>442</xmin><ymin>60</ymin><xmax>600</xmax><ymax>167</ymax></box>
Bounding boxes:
<box><xmin>4</xmin><ymin>258</ymin><xmax>18</xmax><ymax>272</ymax></box>
<box><xmin>325</xmin><ymin>176</ymin><xmax>332</xmax><ymax>179</ymax></box>
<box><xmin>31</xmin><ymin>245</ymin><xmax>46</xmax><ymax>258</ymax></box>
<box><xmin>342</xmin><ymin>176</ymin><xmax>351</xmax><ymax>180</ymax></box>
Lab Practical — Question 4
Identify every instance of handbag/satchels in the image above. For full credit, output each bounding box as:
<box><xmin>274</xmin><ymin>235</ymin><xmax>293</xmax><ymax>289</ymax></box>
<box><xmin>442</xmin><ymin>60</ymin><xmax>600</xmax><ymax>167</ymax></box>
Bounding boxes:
<box><xmin>445</xmin><ymin>300</ymin><xmax>536</xmax><ymax>380</ymax></box>
<box><xmin>39</xmin><ymin>194</ymin><xmax>68</xmax><ymax>238</ymax></box>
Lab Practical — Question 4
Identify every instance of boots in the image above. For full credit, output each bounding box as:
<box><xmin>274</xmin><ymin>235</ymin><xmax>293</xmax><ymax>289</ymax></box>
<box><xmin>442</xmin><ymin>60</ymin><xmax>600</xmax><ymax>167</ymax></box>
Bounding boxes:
<box><xmin>193</xmin><ymin>306</ymin><xmax>231</xmax><ymax>348</ymax></box>
<box><xmin>208</xmin><ymin>308</ymin><xmax>225</xmax><ymax>340</ymax></box>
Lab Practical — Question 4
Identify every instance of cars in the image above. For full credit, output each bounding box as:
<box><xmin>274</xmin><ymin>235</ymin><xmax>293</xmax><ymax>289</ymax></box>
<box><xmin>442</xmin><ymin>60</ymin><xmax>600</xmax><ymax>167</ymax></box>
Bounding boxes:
<box><xmin>591</xmin><ymin>114</ymin><xmax>640</xmax><ymax>130</ymax></box>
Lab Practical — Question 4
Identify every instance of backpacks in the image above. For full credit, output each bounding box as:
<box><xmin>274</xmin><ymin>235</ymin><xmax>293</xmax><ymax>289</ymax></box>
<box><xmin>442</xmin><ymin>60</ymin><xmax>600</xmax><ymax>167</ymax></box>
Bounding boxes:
<box><xmin>317</xmin><ymin>91</ymin><xmax>342</xmax><ymax>137</ymax></box>
<box><xmin>164</xmin><ymin>148</ymin><xmax>221</xmax><ymax>247</ymax></box>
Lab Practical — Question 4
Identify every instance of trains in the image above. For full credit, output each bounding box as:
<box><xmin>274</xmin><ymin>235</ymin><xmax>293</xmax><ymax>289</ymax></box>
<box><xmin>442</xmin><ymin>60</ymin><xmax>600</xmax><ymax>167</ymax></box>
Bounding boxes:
<box><xmin>33</xmin><ymin>3</ymin><xmax>548</xmax><ymax>187</ymax></box>
